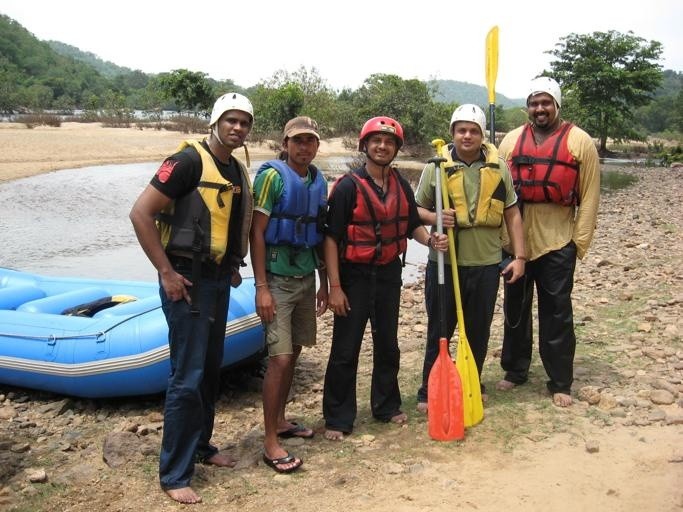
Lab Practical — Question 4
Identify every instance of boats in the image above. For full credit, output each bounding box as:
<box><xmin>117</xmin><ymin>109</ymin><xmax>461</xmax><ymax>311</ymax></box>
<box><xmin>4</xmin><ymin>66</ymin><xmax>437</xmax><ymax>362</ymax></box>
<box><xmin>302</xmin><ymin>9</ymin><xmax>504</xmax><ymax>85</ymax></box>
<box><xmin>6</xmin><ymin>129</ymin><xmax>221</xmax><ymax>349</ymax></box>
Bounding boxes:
<box><xmin>0</xmin><ymin>266</ymin><xmax>266</xmax><ymax>400</ymax></box>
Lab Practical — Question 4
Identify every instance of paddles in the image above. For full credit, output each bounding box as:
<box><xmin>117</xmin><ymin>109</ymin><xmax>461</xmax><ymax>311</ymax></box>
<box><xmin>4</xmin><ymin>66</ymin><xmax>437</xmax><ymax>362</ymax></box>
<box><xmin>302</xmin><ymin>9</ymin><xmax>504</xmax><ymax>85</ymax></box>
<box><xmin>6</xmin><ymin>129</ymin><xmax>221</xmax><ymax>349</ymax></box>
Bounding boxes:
<box><xmin>427</xmin><ymin>139</ymin><xmax>484</xmax><ymax>441</ymax></box>
<box><xmin>485</xmin><ymin>25</ymin><xmax>500</xmax><ymax>147</ymax></box>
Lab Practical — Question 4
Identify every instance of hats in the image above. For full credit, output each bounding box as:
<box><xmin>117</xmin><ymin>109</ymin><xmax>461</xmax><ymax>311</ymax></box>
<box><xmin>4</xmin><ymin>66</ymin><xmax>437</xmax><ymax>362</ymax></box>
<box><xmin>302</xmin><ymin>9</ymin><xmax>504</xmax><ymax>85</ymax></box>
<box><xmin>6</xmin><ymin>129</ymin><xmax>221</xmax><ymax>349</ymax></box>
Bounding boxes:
<box><xmin>284</xmin><ymin>116</ymin><xmax>320</xmax><ymax>140</ymax></box>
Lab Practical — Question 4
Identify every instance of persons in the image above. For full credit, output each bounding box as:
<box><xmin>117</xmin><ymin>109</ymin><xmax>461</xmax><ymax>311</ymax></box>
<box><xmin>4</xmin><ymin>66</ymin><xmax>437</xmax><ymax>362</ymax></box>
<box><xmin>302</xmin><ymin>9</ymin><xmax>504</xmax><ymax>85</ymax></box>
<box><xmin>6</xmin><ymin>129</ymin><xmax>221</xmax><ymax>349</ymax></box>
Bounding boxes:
<box><xmin>128</xmin><ymin>92</ymin><xmax>254</xmax><ymax>505</ymax></box>
<box><xmin>249</xmin><ymin>115</ymin><xmax>330</xmax><ymax>472</ymax></box>
<box><xmin>321</xmin><ymin>116</ymin><xmax>448</xmax><ymax>441</ymax></box>
<box><xmin>414</xmin><ymin>102</ymin><xmax>527</xmax><ymax>414</ymax></box>
<box><xmin>497</xmin><ymin>76</ymin><xmax>601</xmax><ymax>406</ymax></box>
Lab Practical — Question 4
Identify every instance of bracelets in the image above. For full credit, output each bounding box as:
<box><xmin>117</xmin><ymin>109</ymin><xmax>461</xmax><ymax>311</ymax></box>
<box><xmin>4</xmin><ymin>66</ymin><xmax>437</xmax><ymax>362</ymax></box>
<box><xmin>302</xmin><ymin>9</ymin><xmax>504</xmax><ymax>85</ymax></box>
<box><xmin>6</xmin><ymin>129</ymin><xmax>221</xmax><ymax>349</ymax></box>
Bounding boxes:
<box><xmin>511</xmin><ymin>256</ymin><xmax>527</xmax><ymax>261</ymax></box>
<box><xmin>428</xmin><ymin>238</ymin><xmax>431</xmax><ymax>248</ymax></box>
<box><xmin>330</xmin><ymin>285</ymin><xmax>341</xmax><ymax>287</ymax></box>
<box><xmin>255</xmin><ymin>282</ymin><xmax>268</xmax><ymax>287</ymax></box>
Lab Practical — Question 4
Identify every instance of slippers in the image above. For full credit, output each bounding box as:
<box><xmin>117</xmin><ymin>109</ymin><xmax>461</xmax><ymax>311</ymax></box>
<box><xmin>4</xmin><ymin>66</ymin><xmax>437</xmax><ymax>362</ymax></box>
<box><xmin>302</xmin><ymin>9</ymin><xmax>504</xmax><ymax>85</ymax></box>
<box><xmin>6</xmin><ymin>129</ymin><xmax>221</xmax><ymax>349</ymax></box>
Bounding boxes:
<box><xmin>277</xmin><ymin>422</ymin><xmax>313</xmax><ymax>438</ymax></box>
<box><xmin>263</xmin><ymin>451</ymin><xmax>303</xmax><ymax>472</ymax></box>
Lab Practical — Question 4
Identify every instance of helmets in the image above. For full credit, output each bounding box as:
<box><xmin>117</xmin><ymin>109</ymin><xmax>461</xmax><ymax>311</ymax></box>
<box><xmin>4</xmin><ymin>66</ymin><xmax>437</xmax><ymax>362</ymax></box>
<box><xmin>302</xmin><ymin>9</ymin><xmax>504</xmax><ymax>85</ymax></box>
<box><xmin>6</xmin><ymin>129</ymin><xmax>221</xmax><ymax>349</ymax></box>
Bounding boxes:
<box><xmin>359</xmin><ymin>116</ymin><xmax>404</xmax><ymax>151</ymax></box>
<box><xmin>209</xmin><ymin>92</ymin><xmax>254</xmax><ymax>126</ymax></box>
<box><xmin>526</xmin><ymin>77</ymin><xmax>561</xmax><ymax>108</ymax></box>
<box><xmin>449</xmin><ymin>103</ymin><xmax>486</xmax><ymax>136</ymax></box>
